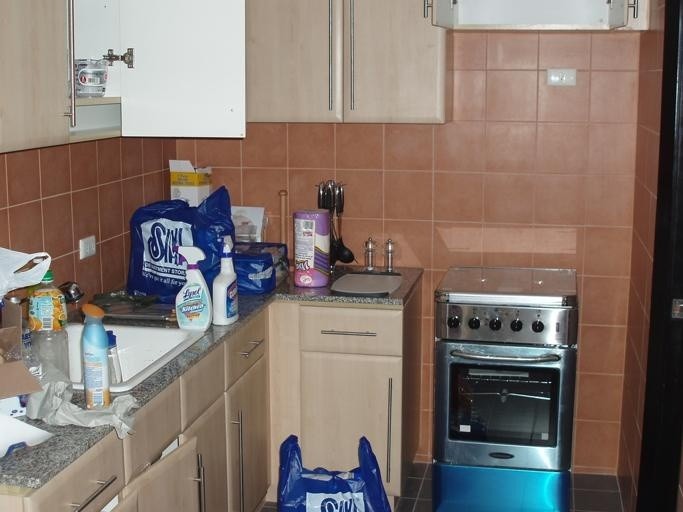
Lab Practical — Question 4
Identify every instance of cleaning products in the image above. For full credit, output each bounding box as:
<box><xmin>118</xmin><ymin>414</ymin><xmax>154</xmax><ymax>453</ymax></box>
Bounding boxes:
<box><xmin>80</xmin><ymin>302</ymin><xmax>111</xmax><ymax>409</ymax></box>
<box><xmin>212</xmin><ymin>234</ymin><xmax>241</xmax><ymax>326</ymax></box>
<box><xmin>172</xmin><ymin>245</ymin><xmax>213</xmax><ymax>332</ymax></box>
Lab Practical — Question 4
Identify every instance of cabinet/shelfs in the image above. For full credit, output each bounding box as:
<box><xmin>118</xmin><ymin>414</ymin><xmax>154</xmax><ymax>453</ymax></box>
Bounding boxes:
<box><xmin>226</xmin><ymin>306</ymin><xmax>271</xmax><ymax>512</ymax></box>
<box><xmin>0</xmin><ymin>0</ymin><xmax>248</xmax><ymax>156</ymax></box>
<box><xmin>419</xmin><ymin>1</ymin><xmax>665</xmax><ymax>35</ymax></box>
<box><xmin>298</xmin><ymin>307</ymin><xmax>423</xmax><ymax>499</ymax></box>
<box><xmin>244</xmin><ymin>2</ymin><xmax>449</xmax><ymax>127</ymax></box>
<box><xmin>120</xmin><ymin>339</ymin><xmax>228</xmax><ymax>511</ymax></box>
<box><xmin>0</xmin><ymin>426</ymin><xmax>139</xmax><ymax>512</ymax></box>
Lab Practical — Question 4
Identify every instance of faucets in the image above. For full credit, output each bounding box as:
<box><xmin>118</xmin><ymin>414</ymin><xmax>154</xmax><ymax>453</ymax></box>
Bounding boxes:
<box><xmin>57</xmin><ymin>281</ymin><xmax>85</xmax><ymax>305</ymax></box>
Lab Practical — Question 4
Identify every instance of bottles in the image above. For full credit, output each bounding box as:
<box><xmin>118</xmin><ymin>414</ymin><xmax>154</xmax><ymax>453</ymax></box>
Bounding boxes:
<box><xmin>102</xmin><ymin>330</ymin><xmax>122</xmax><ymax>384</ymax></box>
<box><xmin>29</xmin><ymin>270</ymin><xmax>69</xmax><ymax>395</ymax></box>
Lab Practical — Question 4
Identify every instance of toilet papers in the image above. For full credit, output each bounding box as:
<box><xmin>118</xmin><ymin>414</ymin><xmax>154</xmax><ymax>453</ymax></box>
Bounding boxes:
<box><xmin>292</xmin><ymin>207</ymin><xmax>331</xmax><ymax>289</ymax></box>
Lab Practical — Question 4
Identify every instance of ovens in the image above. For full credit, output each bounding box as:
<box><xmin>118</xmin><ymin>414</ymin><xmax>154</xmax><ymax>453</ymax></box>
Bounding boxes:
<box><xmin>433</xmin><ymin>304</ymin><xmax>575</xmax><ymax>471</ymax></box>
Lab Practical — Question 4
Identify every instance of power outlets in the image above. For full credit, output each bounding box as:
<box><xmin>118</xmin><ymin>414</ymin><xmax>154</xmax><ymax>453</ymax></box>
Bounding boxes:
<box><xmin>78</xmin><ymin>235</ymin><xmax>97</xmax><ymax>262</ymax></box>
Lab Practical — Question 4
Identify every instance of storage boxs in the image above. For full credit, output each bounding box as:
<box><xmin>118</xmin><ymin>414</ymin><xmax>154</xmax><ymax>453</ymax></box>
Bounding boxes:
<box><xmin>0</xmin><ymin>300</ymin><xmax>43</xmax><ymax>403</ymax></box>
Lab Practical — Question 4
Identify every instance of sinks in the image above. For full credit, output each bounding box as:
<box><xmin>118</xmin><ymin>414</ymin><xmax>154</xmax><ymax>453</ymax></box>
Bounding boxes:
<box><xmin>58</xmin><ymin>321</ymin><xmax>206</xmax><ymax>394</ymax></box>
